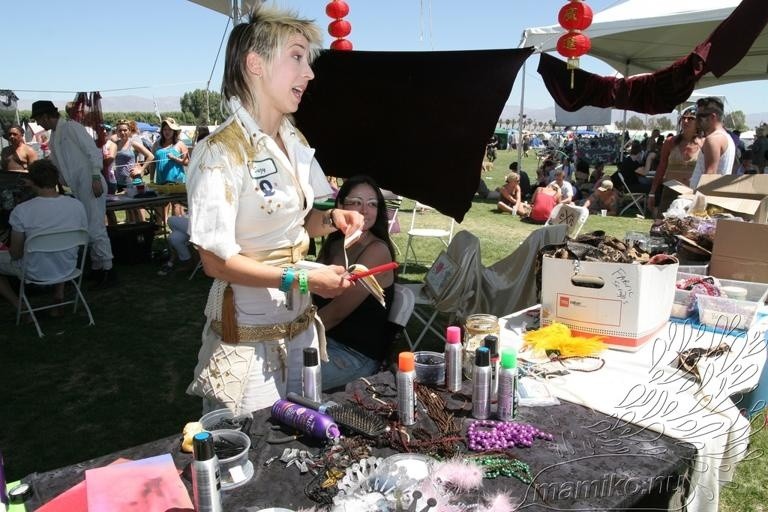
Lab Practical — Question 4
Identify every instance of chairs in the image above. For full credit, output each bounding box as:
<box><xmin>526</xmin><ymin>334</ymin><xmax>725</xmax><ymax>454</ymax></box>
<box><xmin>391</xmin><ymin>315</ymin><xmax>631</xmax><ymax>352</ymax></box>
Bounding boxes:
<box><xmin>402</xmin><ymin>200</ymin><xmax>455</xmax><ymax>274</ymax></box>
<box><xmin>189</xmin><ymin>258</ymin><xmax>203</xmax><ymax>281</ymax></box>
<box><xmin>381</xmin><ymin>280</ymin><xmax>416</xmax><ymax>371</ymax></box>
<box><xmin>403</xmin><ymin>229</ymin><xmax>481</xmax><ymax>351</ymax></box>
<box><xmin>385</xmin><ymin>196</ymin><xmax>403</xmax><ymax>255</ymax></box>
<box><xmin>15</xmin><ymin>228</ymin><xmax>96</xmax><ymax>337</ymax></box>
<box><xmin>618</xmin><ymin>172</ymin><xmax>648</xmax><ymax>219</ymax></box>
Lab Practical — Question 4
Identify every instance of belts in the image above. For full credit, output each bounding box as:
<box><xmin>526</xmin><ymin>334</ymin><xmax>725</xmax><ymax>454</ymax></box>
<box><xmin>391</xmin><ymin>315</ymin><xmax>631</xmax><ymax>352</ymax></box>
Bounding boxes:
<box><xmin>210</xmin><ymin>301</ymin><xmax>317</xmax><ymax>342</ymax></box>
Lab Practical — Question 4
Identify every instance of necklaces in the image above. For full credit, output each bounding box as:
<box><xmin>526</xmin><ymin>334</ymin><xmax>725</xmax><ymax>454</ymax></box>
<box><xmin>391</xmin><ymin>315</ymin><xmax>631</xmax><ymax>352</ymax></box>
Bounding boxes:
<box><xmin>467</xmin><ymin>420</ymin><xmax>552</xmax><ymax>452</ymax></box>
<box><xmin>472</xmin><ymin>457</ymin><xmax>533</xmax><ymax>485</ymax></box>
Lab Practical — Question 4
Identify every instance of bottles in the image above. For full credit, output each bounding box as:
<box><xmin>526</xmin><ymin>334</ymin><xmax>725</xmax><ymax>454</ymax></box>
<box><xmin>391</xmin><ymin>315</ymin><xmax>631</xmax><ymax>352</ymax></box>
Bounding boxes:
<box><xmin>470</xmin><ymin>346</ymin><xmax>492</xmax><ymax>420</ymax></box>
<box><xmin>131</xmin><ymin>179</ymin><xmax>144</xmax><ymax>195</ymax></box>
<box><xmin>189</xmin><ymin>433</ymin><xmax>223</xmax><ymax>512</ymax></box>
<box><xmin>498</xmin><ymin>348</ymin><xmax>520</xmax><ymax>420</ymax></box>
<box><xmin>272</xmin><ymin>399</ymin><xmax>340</xmax><ymax>441</ymax></box>
<box><xmin>302</xmin><ymin>349</ymin><xmax>323</xmax><ymax>404</ymax></box>
<box><xmin>482</xmin><ymin>333</ymin><xmax>499</xmax><ymax>402</ymax></box>
<box><xmin>444</xmin><ymin>326</ymin><xmax>463</xmax><ymax>393</ymax></box>
<box><xmin>394</xmin><ymin>351</ymin><xmax>418</xmax><ymax>426</ymax></box>
<box><xmin>463</xmin><ymin>312</ymin><xmax>501</xmax><ymax>383</ymax></box>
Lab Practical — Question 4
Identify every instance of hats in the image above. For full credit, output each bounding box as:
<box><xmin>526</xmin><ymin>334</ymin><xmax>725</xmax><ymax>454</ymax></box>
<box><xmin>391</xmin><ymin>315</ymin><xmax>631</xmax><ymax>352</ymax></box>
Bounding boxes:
<box><xmin>598</xmin><ymin>180</ymin><xmax>613</xmax><ymax>192</ymax></box>
<box><xmin>30</xmin><ymin>100</ymin><xmax>58</xmax><ymax>119</ymax></box>
<box><xmin>161</xmin><ymin>117</ymin><xmax>182</xmax><ymax>136</ymax></box>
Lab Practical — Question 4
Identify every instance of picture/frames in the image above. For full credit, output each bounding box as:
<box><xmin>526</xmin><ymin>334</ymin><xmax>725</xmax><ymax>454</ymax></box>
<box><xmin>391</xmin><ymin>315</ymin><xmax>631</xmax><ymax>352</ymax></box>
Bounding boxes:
<box><xmin>423</xmin><ymin>250</ymin><xmax>462</xmax><ymax>302</ymax></box>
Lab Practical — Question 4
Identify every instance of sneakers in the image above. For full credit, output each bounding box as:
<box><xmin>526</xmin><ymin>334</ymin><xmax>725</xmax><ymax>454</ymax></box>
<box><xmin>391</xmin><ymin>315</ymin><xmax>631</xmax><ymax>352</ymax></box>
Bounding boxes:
<box><xmin>18</xmin><ymin>257</ymin><xmax>194</xmax><ymax>324</ymax></box>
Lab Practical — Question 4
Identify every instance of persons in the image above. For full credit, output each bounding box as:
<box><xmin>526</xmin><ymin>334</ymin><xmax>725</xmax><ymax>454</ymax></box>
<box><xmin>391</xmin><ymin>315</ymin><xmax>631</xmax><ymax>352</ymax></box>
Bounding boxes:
<box><xmin>0</xmin><ymin>0</ymin><xmax>768</xmax><ymax>434</ymax></box>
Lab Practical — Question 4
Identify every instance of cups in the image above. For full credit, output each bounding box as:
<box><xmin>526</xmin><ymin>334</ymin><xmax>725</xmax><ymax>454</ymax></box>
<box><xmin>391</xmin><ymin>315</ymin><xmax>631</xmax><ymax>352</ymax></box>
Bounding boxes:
<box><xmin>600</xmin><ymin>210</ymin><xmax>608</xmax><ymax>217</ymax></box>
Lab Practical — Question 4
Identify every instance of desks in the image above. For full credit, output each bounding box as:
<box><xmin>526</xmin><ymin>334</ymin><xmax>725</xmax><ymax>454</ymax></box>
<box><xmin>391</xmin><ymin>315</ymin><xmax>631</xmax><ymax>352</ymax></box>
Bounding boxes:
<box><xmin>105</xmin><ymin>182</ymin><xmax>187</xmax><ymax>258</ymax></box>
<box><xmin>499</xmin><ymin>299</ymin><xmax>768</xmax><ymax>510</ymax></box>
<box><xmin>0</xmin><ymin>368</ymin><xmax>698</xmax><ymax>511</ymax></box>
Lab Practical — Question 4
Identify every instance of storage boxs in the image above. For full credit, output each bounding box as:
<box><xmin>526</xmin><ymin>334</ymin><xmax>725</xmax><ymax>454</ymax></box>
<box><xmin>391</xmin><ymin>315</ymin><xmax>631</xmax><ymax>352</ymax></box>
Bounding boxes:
<box><xmin>663</xmin><ymin>173</ymin><xmax>768</xmax><ymax>224</ymax></box>
<box><xmin>540</xmin><ymin>250</ymin><xmax>680</xmax><ymax>353</ymax></box>
<box><xmin>696</xmin><ymin>278</ymin><xmax>768</xmax><ymax>331</ymax></box>
<box><xmin>673</xmin><ymin>218</ymin><xmax>768</xmax><ymax>283</ymax></box>
<box><xmin>678</xmin><ymin>263</ymin><xmax>711</xmax><ymax>275</ymax></box>
<box><xmin>671</xmin><ymin>271</ymin><xmax>709</xmax><ymax>319</ymax></box>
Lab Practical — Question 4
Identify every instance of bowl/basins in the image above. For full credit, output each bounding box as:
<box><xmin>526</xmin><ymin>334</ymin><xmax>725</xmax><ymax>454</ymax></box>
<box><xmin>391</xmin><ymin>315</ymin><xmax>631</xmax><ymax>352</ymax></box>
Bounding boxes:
<box><xmin>198</xmin><ymin>407</ymin><xmax>255</xmax><ymax>481</ymax></box>
<box><xmin>411</xmin><ymin>350</ymin><xmax>447</xmax><ymax>384</ymax></box>
